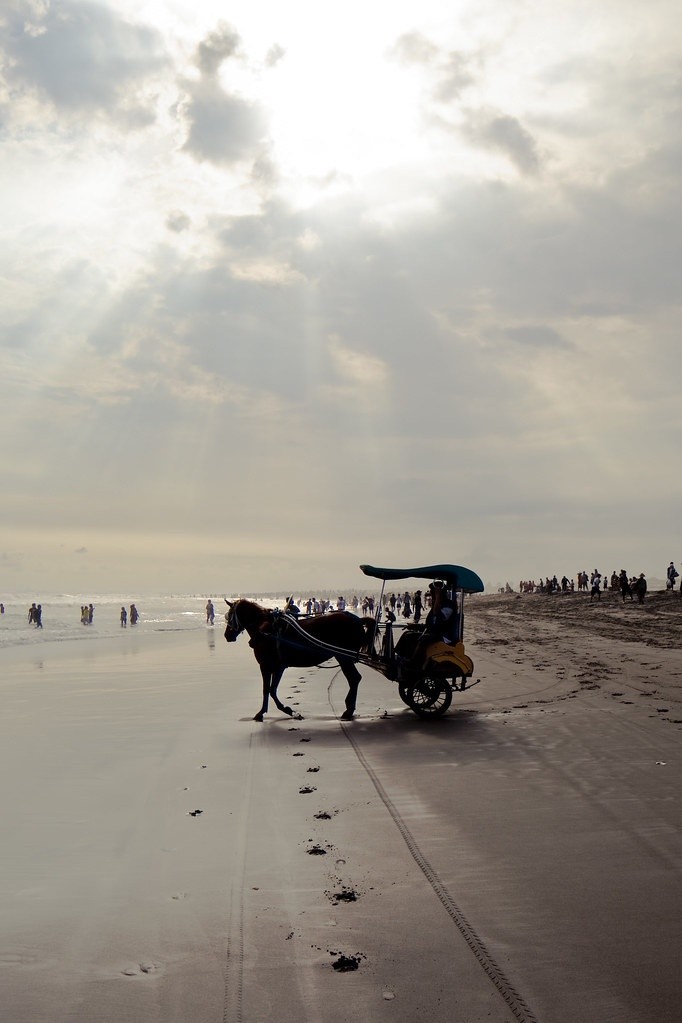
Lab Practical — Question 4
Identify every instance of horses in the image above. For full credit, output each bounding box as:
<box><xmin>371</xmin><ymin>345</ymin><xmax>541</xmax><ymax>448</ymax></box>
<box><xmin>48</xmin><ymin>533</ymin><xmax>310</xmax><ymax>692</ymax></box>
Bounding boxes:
<box><xmin>223</xmin><ymin>599</ymin><xmax>380</xmax><ymax>721</ymax></box>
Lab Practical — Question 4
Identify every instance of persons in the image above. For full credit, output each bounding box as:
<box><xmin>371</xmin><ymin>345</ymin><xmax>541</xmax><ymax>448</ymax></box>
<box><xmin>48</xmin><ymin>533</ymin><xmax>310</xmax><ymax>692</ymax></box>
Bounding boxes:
<box><xmin>28</xmin><ymin>603</ymin><xmax>42</xmax><ymax>628</ymax></box>
<box><xmin>130</xmin><ymin>604</ymin><xmax>139</xmax><ymax>624</ymax></box>
<box><xmin>206</xmin><ymin>600</ymin><xmax>215</xmax><ymax>625</ymax></box>
<box><xmin>0</xmin><ymin>604</ymin><xmax>5</xmax><ymax>614</ymax></box>
<box><xmin>121</xmin><ymin>607</ymin><xmax>127</xmax><ymax>626</ymax></box>
<box><xmin>394</xmin><ymin>581</ymin><xmax>458</xmax><ymax>673</ymax></box>
<box><xmin>297</xmin><ymin>590</ymin><xmax>430</xmax><ymax>624</ymax></box>
<box><xmin>666</xmin><ymin>562</ymin><xmax>682</xmax><ymax>591</ymax></box>
<box><xmin>81</xmin><ymin>604</ymin><xmax>95</xmax><ymax>625</ymax></box>
<box><xmin>500</xmin><ymin>568</ymin><xmax>647</xmax><ymax>605</ymax></box>
<box><xmin>284</xmin><ymin>597</ymin><xmax>301</xmax><ymax>620</ymax></box>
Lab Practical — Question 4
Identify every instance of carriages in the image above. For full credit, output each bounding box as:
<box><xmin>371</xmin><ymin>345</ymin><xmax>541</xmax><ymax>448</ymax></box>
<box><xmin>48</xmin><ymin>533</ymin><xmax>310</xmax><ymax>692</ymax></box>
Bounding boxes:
<box><xmin>223</xmin><ymin>563</ymin><xmax>484</xmax><ymax>723</ymax></box>
